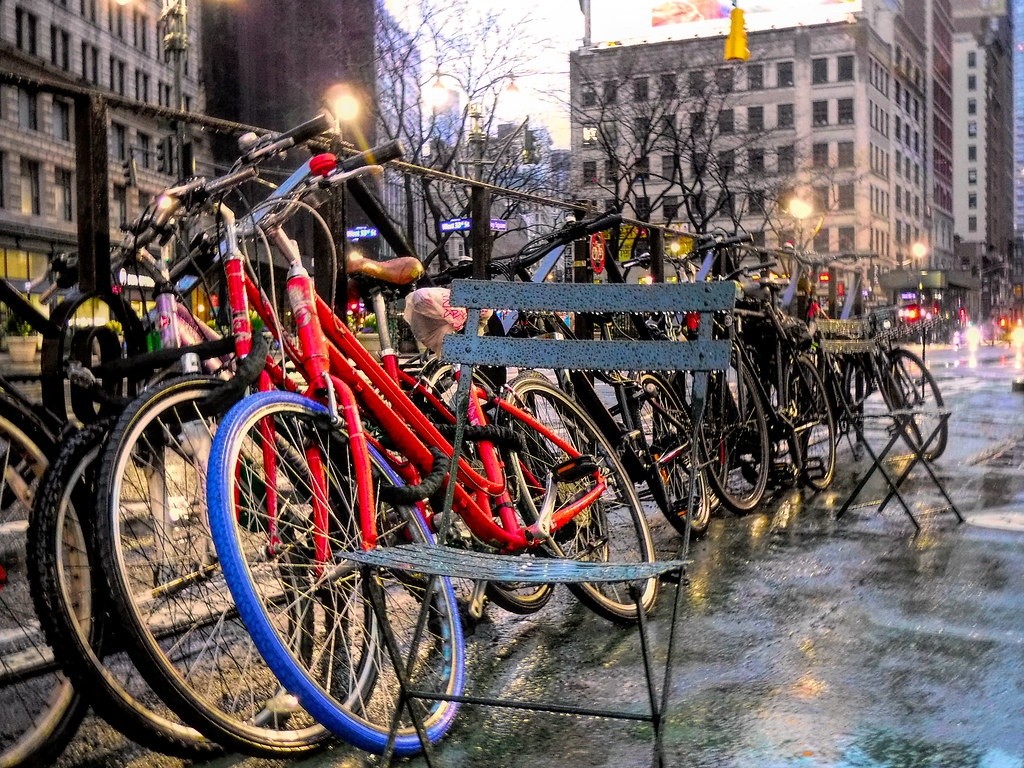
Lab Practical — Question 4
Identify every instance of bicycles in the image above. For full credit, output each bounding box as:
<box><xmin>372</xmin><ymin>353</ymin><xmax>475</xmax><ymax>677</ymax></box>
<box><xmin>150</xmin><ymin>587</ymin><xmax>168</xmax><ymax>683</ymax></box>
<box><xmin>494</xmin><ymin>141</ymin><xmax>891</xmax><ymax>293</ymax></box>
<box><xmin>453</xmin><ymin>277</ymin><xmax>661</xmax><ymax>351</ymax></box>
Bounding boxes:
<box><xmin>0</xmin><ymin>110</ymin><xmax>952</xmax><ymax>768</ymax></box>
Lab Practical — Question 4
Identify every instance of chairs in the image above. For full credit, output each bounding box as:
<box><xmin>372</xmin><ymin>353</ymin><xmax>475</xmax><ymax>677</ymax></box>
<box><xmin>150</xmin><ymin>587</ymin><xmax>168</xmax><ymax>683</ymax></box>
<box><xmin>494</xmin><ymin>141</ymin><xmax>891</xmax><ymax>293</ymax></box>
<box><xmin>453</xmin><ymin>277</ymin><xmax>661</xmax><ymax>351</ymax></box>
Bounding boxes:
<box><xmin>816</xmin><ymin>318</ymin><xmax>965</xmax><ymax>531</ymax></box>
<box><xmin>336</xmin><ymin>279</ymin><xmax>737</xmax><ymax>768</ymax></box>
